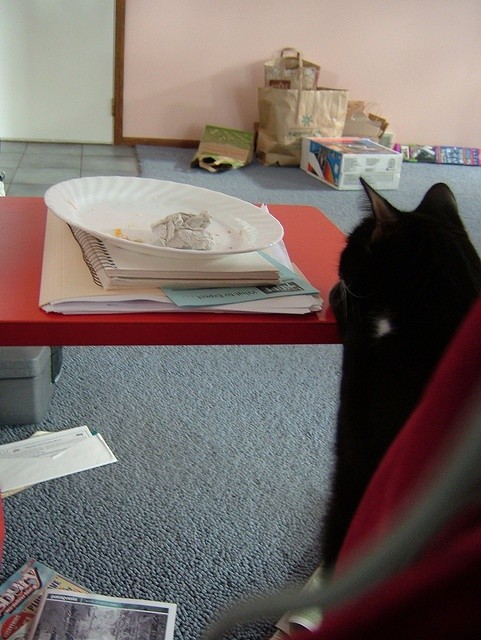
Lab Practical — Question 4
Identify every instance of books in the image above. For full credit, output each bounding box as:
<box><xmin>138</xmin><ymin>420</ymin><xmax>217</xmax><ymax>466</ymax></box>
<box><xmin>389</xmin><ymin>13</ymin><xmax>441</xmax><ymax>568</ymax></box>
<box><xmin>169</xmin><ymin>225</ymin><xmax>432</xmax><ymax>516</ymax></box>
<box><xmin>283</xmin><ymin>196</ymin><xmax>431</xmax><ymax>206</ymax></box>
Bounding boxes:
<box><xmin>26</xmin><ymin>587</ymin><xmax>179</xmax><ymax>640</ymax></box>
<box><xmin>66</xmin><ymin>221</ymin><xmax>284</xmax><ymax>292</ymax></box>
<box><xmin>1</xmin><ymin>556</ymin><xmax>101</xmax><ymax>640</ymax></box>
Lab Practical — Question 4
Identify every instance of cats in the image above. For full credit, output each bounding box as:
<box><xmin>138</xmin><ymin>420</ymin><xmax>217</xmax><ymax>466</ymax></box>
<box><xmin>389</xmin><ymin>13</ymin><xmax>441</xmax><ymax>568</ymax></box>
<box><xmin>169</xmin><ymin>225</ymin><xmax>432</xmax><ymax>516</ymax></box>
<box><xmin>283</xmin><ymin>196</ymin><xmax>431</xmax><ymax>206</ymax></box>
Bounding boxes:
<box><xmin>317</xmin><ymin>176</ymin><xmax>481</xmax><ymax>583</ymax></box>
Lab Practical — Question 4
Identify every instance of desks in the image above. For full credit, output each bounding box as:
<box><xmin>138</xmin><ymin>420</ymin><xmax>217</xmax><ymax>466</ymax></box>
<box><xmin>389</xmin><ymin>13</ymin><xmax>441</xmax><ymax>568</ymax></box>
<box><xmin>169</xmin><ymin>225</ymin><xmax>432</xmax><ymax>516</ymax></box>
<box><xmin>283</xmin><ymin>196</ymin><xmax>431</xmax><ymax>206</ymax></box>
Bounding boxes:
<box><xmin>0</xmin><ymin>196</ymin><xmax>350</xmax><ymax>347</ymax></box>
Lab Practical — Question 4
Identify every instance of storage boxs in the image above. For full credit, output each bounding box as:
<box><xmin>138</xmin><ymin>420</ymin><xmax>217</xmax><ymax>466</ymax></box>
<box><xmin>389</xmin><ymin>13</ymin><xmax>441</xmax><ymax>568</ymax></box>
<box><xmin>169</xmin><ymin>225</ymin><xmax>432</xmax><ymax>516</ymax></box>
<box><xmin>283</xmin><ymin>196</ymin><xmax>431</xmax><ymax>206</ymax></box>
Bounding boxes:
<box><xmin>300</xmin><ymin>136</ymin><xmax>403</xmax><ymax>191</ymax></box>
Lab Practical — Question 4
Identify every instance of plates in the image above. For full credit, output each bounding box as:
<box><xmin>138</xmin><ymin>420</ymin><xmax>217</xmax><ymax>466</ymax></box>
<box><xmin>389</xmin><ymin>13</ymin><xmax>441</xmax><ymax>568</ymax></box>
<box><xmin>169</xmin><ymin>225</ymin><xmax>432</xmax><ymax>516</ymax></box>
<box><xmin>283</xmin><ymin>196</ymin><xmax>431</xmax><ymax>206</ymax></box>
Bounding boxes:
<box><xmin>44</xmin><ymin>176</ymin><xmax>284</xmax><ymax>259</ymax></box>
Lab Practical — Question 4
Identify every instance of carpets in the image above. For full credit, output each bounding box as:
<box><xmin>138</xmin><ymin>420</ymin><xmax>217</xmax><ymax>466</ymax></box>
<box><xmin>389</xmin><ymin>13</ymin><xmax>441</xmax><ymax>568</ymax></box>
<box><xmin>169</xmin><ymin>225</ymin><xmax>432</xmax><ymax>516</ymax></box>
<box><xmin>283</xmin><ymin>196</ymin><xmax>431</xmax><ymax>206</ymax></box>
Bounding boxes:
<box><xmin>1</xmin><ymin>144</ymin><xmax>481</xmax><ymax>639</ymax></box>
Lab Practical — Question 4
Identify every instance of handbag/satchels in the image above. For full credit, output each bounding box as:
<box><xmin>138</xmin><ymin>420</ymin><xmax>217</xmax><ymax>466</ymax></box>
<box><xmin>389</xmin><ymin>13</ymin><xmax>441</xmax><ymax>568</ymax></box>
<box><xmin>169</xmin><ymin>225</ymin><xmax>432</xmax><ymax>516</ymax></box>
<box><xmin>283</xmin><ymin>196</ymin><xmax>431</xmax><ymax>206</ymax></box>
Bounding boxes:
<box><xmin>264</xmin><ymin>48</ymin><xmax>321</xmax><ymax>90</ymax></box>
<box><xmin>343</xmin><ymin>102</ymin><xmax>384</xmax><ymax>137</ymax></box>
<box><xmin>255</xmin><ymin>75</ymin><xmax>349</xmax><ymax>165</ymax></box>
<box><xmin>191</xmin><ymin>125</ymin><xmax>254</xmax><ymax>173</ymax></box>
